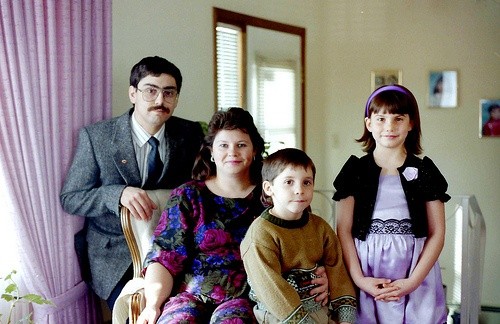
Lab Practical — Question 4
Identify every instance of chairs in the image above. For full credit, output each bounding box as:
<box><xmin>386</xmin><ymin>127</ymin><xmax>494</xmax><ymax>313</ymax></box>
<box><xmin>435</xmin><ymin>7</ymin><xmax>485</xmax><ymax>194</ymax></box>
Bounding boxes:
<box><xmin>120</xmin><ymin>188</ymin><xmax>185</xmax><ymax>324</ymax></box>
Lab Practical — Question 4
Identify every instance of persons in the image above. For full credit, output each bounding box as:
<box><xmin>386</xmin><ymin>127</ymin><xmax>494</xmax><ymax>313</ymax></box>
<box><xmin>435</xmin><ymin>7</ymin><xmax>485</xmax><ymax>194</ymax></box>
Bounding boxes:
<box><xmin>59</xmin><ymin>56</ymin><xmax>208</xmax><ymax>324</ymax></box>
<box><xmin>332</xmin><ymin>84</ymin><xmax>451</xmax><ymax>324</ymax></box>
<box><xmin>240</xmin><ymin>148</ymin><xmax>357</xmax><ymax>324</ymax></box>
<box><xmin>482</xmin><ymin>104</ymin><xmax>500</xmax><ymax>137</ymax></box>
<box><xmin>136</xmin><ymin>107</ymin><xmax>328</xmax><ymax>324</ymax></box>
<box><xmin>433</xmin><ymin>76</ymin><xmax>452</xmax><ymax>106</ymax></box>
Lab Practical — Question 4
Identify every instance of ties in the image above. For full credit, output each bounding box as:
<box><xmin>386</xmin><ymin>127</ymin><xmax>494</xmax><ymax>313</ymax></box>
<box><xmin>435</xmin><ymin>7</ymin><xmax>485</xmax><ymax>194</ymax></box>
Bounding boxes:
<box><xmin>142</xmin><ymin>136</ymin><xmax>164</xmax><ymax>189</ymax></box>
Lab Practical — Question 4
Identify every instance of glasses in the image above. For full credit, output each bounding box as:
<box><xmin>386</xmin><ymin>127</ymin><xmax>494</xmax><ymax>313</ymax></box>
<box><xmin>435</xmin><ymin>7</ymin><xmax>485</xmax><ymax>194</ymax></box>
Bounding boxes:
<box><xmin>136</xmin><ymin>87</ymin><xmax>180</xmax><ymax>104</ymax></box>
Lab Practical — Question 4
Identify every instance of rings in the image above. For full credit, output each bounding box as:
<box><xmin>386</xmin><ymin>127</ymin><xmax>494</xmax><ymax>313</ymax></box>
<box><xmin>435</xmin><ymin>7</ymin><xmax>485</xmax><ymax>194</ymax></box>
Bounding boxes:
<box><xmin>326</xmin><ymin>290</ymin><xmax>329</xmax><ymax>296</ymax></box>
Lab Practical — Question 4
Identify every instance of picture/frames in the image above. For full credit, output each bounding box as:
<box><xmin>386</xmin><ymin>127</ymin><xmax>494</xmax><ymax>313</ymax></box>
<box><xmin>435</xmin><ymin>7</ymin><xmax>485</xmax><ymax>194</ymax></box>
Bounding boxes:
<box><xmin>370</xmin><ymin>69</ymin><xmax>402</xmax><ymax>94</ymax></box>
<box><xmin>424</xmin><ymin>66</ymin><xmax>461</xmax><ymax>110</ymax></box>
<box><xmin>479</xmin><ymin>98</ymin><xmax>500</xmax><ymax>139</ymax></box>
